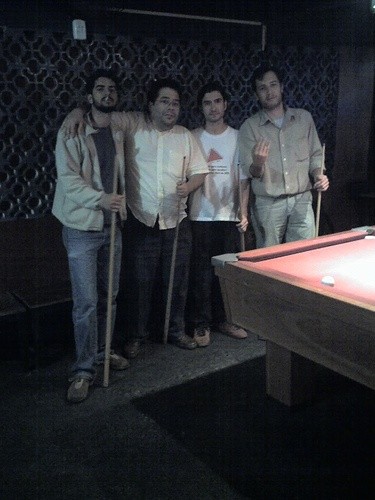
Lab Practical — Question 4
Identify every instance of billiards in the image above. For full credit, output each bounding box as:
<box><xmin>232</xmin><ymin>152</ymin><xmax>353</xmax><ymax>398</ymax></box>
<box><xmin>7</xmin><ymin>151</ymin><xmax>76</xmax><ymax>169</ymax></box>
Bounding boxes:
<box><xmin>321</xmin><ymin>275</ymin><xmax>335</xmax><ymax>287</ymax></box>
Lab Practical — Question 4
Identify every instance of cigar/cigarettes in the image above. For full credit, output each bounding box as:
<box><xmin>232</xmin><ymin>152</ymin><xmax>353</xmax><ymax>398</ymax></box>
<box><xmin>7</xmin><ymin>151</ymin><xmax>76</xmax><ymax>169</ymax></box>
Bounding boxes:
<box><xmin>104</xmin><ymin>95</ymin><xmax>111</xmax><ymax>102</ymax></box>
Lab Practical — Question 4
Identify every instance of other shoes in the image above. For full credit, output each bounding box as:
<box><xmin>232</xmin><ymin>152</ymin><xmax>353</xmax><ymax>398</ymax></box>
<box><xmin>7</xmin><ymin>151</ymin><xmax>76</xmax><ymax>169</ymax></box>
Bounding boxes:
<box><xmin>217</xmin><ymin>320</ymin><xmax>248</xmax><ymax>338</ymax></box>
<box><xmin>96</xmin><ymin>350</ymin><xmax>129</xmax><ymax>371</ymax></box>
<box><xmin>170</xmin><ymin>332</ymin><xmax>199</xmax><ymax>350</ymax></box>
<box><xmin>126</xmin><ymin>340</ymin><xmax>144</xmax><ymax>357</ymax></box>
<box><xmin>195</xmin><ymin>326</ymin><xmax>210</xmax><ymax>347</ymax></box>
<box><xmin>68</xmin><ymin>378</ymin><xmax>91</xmax><ymax>402</ymax></box>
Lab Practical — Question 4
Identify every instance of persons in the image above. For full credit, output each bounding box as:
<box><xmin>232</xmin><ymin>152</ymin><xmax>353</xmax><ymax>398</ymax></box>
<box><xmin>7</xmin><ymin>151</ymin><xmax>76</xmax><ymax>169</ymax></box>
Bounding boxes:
<box><xmin>188</xmin><ymin>82</ymin><xmax>250</xmax><ymax>349</ymax></box>
<box><xmin>49</xmin><ymin>66</ymin><xmax>131</xmax><ymax>404</ymax></box>
<box><xmin>235</xmin><ymin>62</ymin><xmax>330</xmax><ymax>250</ymax></box>
<box><xmin>60</xmin><ymin>77</ymin><xmax>210</xmax><ymax>359</ymax></box>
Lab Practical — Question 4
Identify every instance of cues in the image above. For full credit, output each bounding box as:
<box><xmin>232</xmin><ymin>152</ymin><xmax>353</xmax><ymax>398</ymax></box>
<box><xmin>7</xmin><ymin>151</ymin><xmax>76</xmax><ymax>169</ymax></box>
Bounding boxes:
<box><xmin>103</xmin><ymin>159</ymin><xmax>119</xmax><ymax>387</ymax></box>
<box><xmin>237</xmin><ymin>163</ymin><xmax>245</xmax><ymax>251</ymax></box>
<box><xmin>161</xmin><ymin>156</ymin><xmax>187</xmax><ymax>345</ymax></box>
<box><xmin>315</xmin><ymin>143</ymin><xmax>326</xmax><ymax>237</ymax></box>
